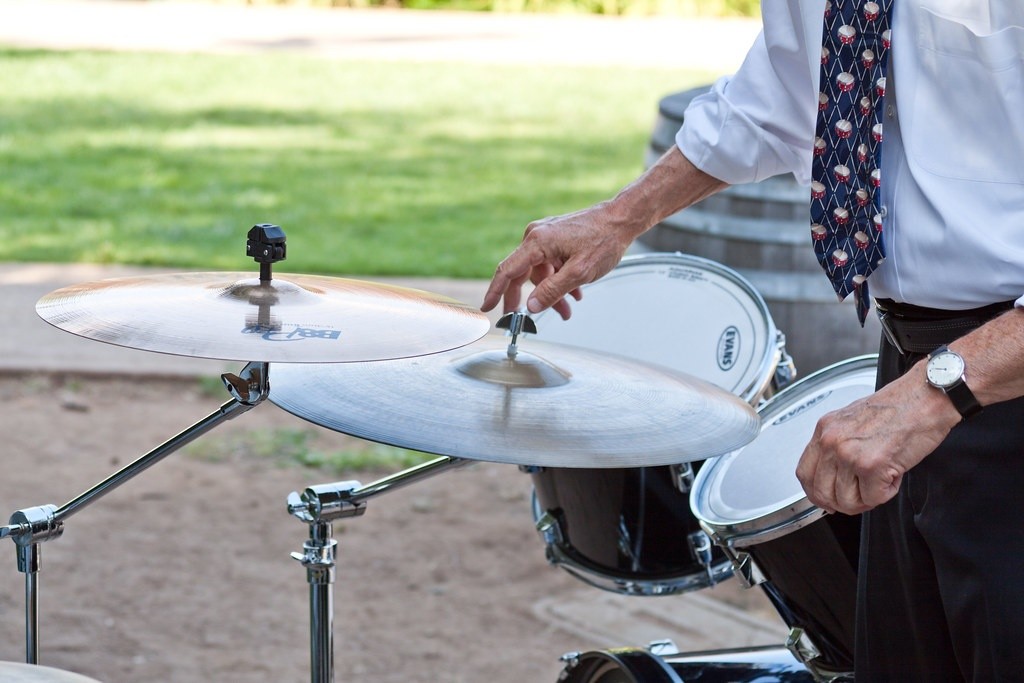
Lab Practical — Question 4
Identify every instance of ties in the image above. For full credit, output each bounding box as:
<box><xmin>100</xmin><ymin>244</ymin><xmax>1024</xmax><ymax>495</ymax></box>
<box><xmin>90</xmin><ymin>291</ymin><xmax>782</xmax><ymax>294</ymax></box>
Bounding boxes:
<box><xmin>810</xmin><ymin>0</ymin><xmax>895</xmax><ymax>304</ymax></box>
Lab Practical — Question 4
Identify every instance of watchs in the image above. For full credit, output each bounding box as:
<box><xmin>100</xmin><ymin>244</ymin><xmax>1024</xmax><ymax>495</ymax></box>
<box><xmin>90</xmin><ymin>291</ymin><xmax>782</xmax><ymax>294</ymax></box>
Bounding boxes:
<box><xmin>926</xmin><ymin>344</ymin><xmax>984</xmax><ymax>422</ymax></box>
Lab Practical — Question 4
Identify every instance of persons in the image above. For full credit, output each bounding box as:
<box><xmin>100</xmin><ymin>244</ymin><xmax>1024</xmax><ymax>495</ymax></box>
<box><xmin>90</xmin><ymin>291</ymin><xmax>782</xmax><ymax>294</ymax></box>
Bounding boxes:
<box><xmin>480</xmin><ymin>0</ymin><xmax>1024</xmax><ymax>683</ymax></box>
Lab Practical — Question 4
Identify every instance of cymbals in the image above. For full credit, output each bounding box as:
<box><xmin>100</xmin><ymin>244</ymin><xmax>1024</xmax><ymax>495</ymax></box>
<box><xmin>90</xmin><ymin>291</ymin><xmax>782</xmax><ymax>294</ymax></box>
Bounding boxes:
<box><xmin>264</xmin><ymin>336</ymin><xmax>761</xmax><ymax>469</ymax></box>
<box><xmin>34</xmin><ymin>268</ymin><xmax>494</xmax><ymax>364</ymax></box>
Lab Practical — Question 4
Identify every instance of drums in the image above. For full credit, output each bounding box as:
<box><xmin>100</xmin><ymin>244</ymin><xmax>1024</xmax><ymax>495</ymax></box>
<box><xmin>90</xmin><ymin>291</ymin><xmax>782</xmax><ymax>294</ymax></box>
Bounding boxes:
<box><xmin>689</xmin><ymin>354</ymin><xmax>881</xmax><ymax>674</ymax></box>
<box><xmin>514</xmin><ymin>252</ymin><xmax>795</xmax><ymax>598</ymax></box>
<box><xmin>555</xmin><ymin>643</ymin><xmax>820</xmax><ymax>682</ymax></box>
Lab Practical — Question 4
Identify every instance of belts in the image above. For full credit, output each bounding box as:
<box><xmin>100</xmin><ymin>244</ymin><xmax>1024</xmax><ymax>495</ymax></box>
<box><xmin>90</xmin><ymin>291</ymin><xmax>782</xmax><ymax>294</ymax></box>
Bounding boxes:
<box><xmin>872</xmin><ymin>297</ymin><xmax>1016</xmax><ymax>355</ymax></box>
